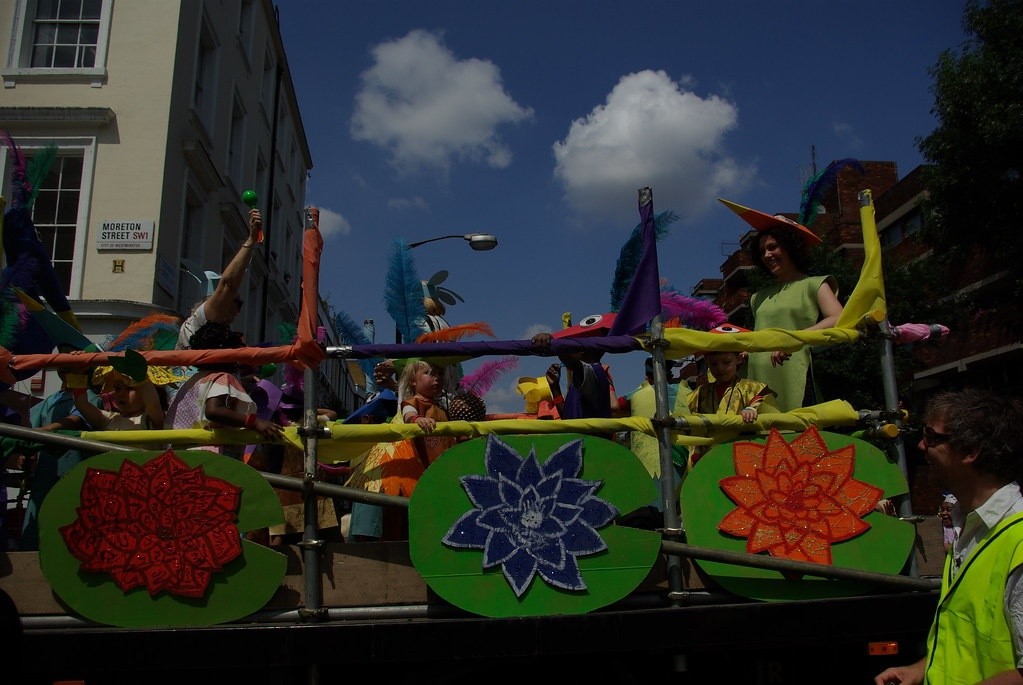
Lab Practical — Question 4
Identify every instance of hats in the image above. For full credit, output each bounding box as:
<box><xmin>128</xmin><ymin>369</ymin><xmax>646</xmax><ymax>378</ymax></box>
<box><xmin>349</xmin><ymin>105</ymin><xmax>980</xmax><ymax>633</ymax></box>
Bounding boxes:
<box><xmin>943</xmin><ymin>493</ymin><xmax>958</xmax><ymax>505</ymax></box>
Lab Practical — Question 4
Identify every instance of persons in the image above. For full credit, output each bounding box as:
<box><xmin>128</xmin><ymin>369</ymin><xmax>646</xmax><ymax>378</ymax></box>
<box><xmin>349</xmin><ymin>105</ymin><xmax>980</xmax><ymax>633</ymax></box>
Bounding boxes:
<box><xmin>0</xmin><ymin>208</ymin><xmax>465</xmax><ymax>552</ymax></box>
<box><xmin>937</xmin><ymin>492</ymin><xmax>960</xmax><ymax>554</ymax></box>
<box><xmin>874</xmin><ymin>386</ymin><xmax>1023</xmax><ymax>685</ymax></box>
<box><xmin>532</xmin><ymin>199</ymin><xmax>844</xmax><ymax>531</ymax></box>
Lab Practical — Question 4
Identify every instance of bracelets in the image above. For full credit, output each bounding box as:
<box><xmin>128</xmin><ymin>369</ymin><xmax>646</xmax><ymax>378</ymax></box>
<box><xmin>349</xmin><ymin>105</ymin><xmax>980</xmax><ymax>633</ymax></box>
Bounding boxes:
<box><xmin>245</xmin><ymin>412</ymin><xmax>257</xmax><ymax>429</ymax></box>
<box><xmin>242</xmin><ymin>244</ymin><xmax>253</xmax><ymax>248</ymax></box>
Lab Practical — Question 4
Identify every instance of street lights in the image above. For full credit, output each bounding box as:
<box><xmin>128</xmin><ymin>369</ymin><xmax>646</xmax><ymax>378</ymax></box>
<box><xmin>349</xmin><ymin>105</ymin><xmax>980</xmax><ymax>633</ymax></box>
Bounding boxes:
<box><xmin>392</xmin><ymin>232</ymin><xmax>498</xmax><ymax>397</ymax></box>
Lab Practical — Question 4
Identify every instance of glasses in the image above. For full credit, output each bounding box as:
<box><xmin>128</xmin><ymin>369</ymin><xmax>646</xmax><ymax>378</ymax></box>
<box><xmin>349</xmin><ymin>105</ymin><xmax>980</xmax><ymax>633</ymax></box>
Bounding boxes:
<box><xmin>923</xmin><ymin>425</ymin><xmax>952</xmax><ymax>449</ymax></box>
